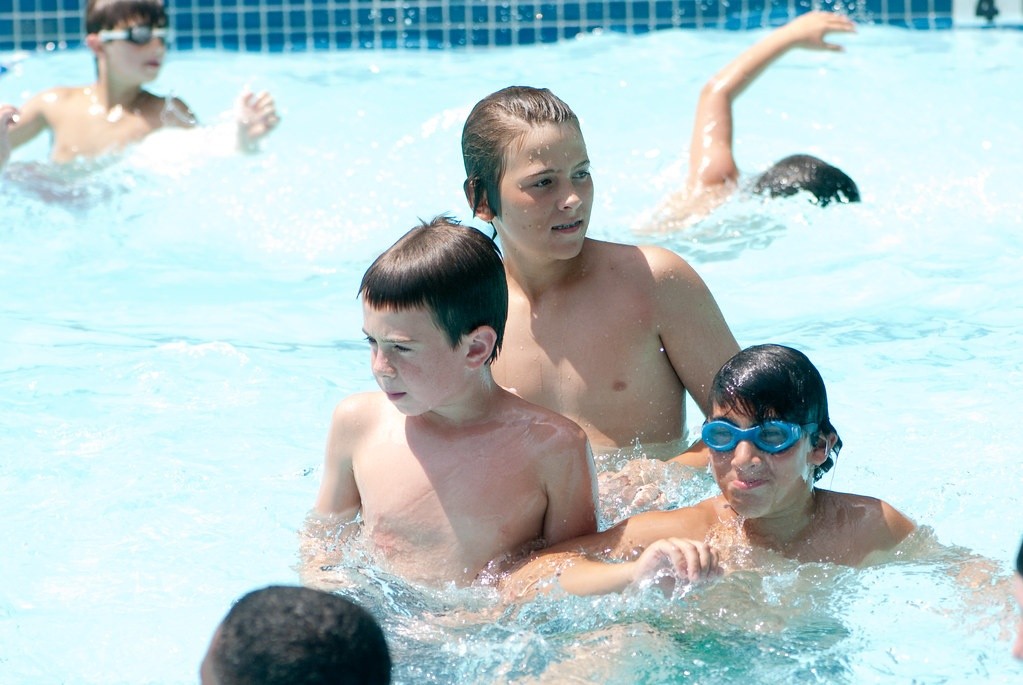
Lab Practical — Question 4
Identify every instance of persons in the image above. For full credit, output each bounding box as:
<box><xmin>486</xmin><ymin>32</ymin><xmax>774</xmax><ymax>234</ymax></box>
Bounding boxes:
<box><xmin>301</xmin><ymin>209</ymin><xmax>598</xmax><ymax>652</ymax></box>
<box><xmin>196</xmin><ymin>583</ymin><xmax>675</xmax><ymax>685</ymax></box>
<box><xmin>628</xmin><ymin>8</ymin><xmax>863</xmax><ymax>238</ymax></box>
<box><xmin>461</xmin><ymin>83</ymin><xmax>745</xmax><ymax>520</ymax></box>
<box><xmin>497</xmin><ymin>339</ymin><xmax>1022</xmax><ymax>640</ymax></box>
<box><xmin>0</xmin><ymin>0</ymin><xmax>281</xmax><ymax>202</ymax></box>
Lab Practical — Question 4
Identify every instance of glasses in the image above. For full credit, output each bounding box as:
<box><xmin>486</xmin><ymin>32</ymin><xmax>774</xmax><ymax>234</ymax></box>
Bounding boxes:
<box><xmin>700</xmin><ymin>420</ymin><xmax>817</xmax><ymax>453</ymax></box>
<box><xmin>100</xmin><ymin>23</ymin><xmax>175</xmax><ymax>47</ymax></box>
